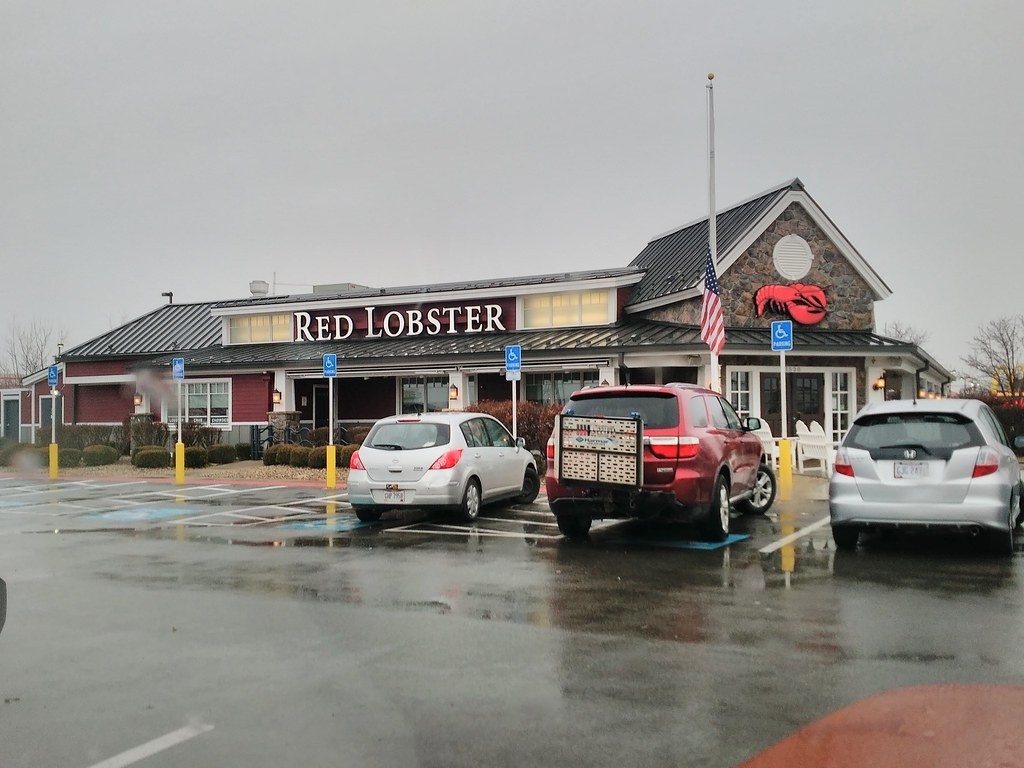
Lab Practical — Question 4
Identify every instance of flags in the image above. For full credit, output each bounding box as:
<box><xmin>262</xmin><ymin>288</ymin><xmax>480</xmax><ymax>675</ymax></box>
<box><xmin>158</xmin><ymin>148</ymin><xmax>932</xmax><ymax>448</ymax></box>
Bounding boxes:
<box><xmin>700</xmin><ymin>242</ymin><xmax>725</xmax><ymax>356</ymax></box>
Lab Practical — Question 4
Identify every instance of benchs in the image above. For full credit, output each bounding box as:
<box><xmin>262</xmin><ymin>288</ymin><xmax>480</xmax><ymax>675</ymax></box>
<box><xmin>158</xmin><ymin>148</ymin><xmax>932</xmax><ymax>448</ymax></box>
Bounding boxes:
<box><xmin>743</xmin><ymin>417</ymin><xmax>779</xmax><ymax>471</ymax></box>
<box><xmin>795</xmin><ymin>420</ymin><xmax>838</xmax><ymax>478</ymax></box>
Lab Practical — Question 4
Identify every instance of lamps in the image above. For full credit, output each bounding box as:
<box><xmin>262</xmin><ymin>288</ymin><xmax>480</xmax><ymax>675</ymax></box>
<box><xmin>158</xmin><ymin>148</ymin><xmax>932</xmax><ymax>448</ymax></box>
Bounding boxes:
<box><xmin>272</xmin><ymin>389</ymin><xmax>281</xmax><ymax>404</ymax></box>
<box><xmin>873</xmin><ymin>376</ymin><xmax>885</xmax><ymax>390</ymax></box>
<box><xmin>450</xmin><ymin>383</ymin><xmax>458</xmax><ymax>400</ymax></box>
<box><xmin>601</xmin><ymin>379</ymin><xmax>609</xmax><ymax>386</ymax></box>
<box><xmin>133</xmin><ymin>391</ymin><xmax>142</xmax><ymax>406</ymax></box>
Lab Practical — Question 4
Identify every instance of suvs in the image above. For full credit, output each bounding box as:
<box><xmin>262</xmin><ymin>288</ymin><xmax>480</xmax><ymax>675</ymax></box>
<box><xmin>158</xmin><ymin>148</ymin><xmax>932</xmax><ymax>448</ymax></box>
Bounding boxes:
<box><xmin>544</xmin><ymin>379</ymin><xmax>777</xmax><ymax>544</ymax></box>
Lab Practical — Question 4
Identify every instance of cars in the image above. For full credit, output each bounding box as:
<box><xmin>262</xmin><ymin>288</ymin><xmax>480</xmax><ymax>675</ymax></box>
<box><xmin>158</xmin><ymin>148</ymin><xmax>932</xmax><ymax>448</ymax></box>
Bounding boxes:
<box><xmin>346</xmin><ymin>411</ymin><xmax>539</xmax><ymax>524</ymax></box>
<box><xmin>828</xmin><ymin>396</ymin><xmax>1024</xmax><ymax>559</ymax></box>
